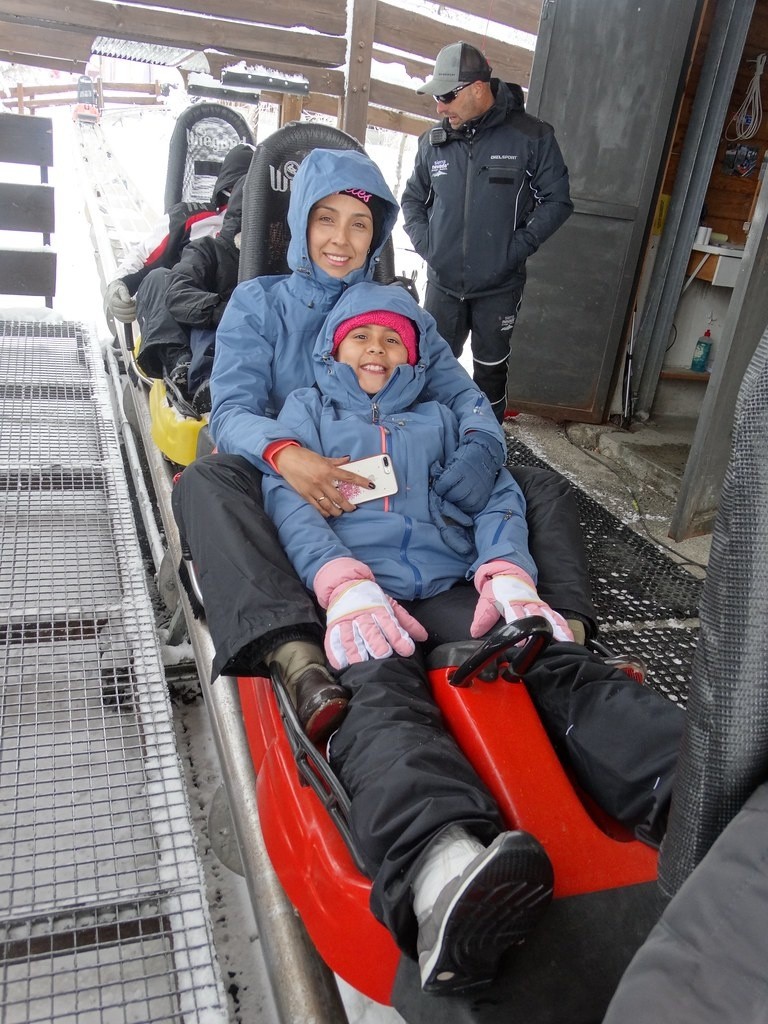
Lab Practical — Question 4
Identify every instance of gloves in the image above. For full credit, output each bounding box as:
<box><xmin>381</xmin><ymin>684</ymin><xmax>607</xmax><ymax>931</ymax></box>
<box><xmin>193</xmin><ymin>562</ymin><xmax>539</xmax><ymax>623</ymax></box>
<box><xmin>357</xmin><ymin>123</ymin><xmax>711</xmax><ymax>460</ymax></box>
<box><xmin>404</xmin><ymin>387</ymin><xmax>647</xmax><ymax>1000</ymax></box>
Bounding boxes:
<box><xmin>469</xmin><ymin>560</ymin><xmax>574</xmax><ymax>646</ymax></box>
<box><xmin>312</xmin><ymin>555</ymin><xmax>429</xmax><ymax>670</ymax></box>
<box><xmin>107</xmin><ymin>282</ymin><xmax>138</xmax><ymax>323</ymax></box>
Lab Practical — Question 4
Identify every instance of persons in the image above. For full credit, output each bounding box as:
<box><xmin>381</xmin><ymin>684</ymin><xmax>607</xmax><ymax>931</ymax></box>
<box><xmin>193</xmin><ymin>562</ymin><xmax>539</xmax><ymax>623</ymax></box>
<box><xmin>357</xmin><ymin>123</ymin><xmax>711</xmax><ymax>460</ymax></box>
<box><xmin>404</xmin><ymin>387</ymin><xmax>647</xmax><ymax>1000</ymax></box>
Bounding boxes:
<box><xmin>401</xmin><ymin>40</ymin><xmax>575</xmax><ymax>427</ymax></box>
<box><xmin>262</xmin><ymin>282</ymin><xmax>686</xmax><ymax>996</ymax></box>
<box><xmin>171</xmin><ymin>147</ymin><xmax>644</xmax><ymax>740</ymax></box>
<box><xmin>165</xmin><ymin>173</ymin><xmax>247</xmax><ymax>414</ymax></box>
<box><xmin>105</xmin><ymin>144</ymin><xmax>256</xmax><ymax>402</ymax></box>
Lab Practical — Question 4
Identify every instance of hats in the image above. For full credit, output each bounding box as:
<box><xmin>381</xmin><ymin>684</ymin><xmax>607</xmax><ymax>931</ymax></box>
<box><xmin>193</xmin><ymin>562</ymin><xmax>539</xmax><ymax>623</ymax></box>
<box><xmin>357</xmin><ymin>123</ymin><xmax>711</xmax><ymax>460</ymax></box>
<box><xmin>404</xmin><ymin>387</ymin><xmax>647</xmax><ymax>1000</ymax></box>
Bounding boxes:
<box><xmin>415</xmin><ymin>41</ymin><xmax>492</xmax><ymax>95</ymax></box>
<box><xmin>332</xmin><ymin>311</ymin><xmax>420</xmax><ymax>369</ymax></box>
<box><xmin>339</xmin><ymin>186</ymin><xmax>382</xmax><ymax>249</ymax></box>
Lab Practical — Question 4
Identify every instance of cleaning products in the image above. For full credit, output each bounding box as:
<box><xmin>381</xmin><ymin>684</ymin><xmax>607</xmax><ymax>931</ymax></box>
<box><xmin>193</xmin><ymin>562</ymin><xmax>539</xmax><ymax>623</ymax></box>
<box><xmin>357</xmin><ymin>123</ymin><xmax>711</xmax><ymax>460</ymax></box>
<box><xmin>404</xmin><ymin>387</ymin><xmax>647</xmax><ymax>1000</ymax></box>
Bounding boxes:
<box><xmin>691</xmin><ymin>329</ymin><xmax>714</xmax><ymax>372</ymax></box>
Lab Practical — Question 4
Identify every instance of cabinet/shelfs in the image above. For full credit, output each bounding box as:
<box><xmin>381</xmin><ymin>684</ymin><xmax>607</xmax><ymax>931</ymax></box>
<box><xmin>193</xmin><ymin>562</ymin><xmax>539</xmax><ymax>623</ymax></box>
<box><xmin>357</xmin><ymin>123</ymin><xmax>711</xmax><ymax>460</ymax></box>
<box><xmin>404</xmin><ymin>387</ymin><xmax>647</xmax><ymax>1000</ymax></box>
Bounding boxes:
<box><xmin>656</xmin><ymin>237</ymin><xmax>754</xmax><ymax>380</ymax></box>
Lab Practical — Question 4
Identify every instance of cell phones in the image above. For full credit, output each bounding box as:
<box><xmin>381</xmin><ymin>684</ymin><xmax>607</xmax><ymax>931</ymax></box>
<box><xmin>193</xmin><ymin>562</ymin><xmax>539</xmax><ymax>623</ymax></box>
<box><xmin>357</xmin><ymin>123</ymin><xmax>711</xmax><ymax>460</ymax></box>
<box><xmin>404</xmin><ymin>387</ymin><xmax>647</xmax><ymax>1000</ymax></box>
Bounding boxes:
<box><xmin>328</xmin><ymin>454</ymin><xmax>399</xmax><ymax>509</ymax></box>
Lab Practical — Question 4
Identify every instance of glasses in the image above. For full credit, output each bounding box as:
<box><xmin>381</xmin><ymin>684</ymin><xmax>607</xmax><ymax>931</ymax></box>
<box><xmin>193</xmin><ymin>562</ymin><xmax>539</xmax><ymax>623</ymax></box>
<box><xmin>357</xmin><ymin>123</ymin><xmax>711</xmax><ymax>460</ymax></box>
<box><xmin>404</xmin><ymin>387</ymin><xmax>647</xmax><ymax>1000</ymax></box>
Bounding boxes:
<box><xmin>432</xmin><ymin>80</ymin><xmax>475</xmax><ymax>105</ymax></box>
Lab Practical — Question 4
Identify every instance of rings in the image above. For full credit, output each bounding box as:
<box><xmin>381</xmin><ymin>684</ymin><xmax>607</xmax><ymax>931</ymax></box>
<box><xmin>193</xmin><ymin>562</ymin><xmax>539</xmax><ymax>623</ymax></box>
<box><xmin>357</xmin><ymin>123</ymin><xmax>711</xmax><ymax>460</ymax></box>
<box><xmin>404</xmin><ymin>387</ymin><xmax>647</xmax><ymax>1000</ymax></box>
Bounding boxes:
<box><xmin>317</xmin><ymin>496</ymin><xmax>325</xmax><ymax>503</ymax></box>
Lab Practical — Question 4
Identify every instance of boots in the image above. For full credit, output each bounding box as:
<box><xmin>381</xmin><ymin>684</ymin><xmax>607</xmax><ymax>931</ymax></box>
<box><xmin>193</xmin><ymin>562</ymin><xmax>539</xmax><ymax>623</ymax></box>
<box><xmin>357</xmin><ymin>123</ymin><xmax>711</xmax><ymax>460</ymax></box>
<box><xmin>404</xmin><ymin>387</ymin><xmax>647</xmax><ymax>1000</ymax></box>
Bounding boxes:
<box><xmin>405</xmin><ymin>823</ymin><xmax>552</xmax><ymax>996</ymax></box>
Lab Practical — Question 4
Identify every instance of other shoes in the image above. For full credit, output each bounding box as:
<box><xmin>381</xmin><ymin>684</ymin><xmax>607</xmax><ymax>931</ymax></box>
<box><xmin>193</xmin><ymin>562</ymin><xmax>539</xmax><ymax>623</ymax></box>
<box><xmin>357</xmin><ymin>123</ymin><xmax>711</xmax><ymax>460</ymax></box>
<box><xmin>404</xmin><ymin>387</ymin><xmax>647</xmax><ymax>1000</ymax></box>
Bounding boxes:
<box><xmin>600</xmin><ymin>655</ymin><xmax>646</xmax><ymax>684</ymax></box>
<box><xmin>293</xmin><ymin>669</ymin><xmax>348</xmax><ymax>742</ymax></box>
<box><xmin>169</xmin><ymin>361</ymin><xmax>193</xmax><ymax>400</ymax></box>
<box><xmin>194</xmin><ymin>379</ymin><xmax>212</xmax><ymax>414</ymax></box>
